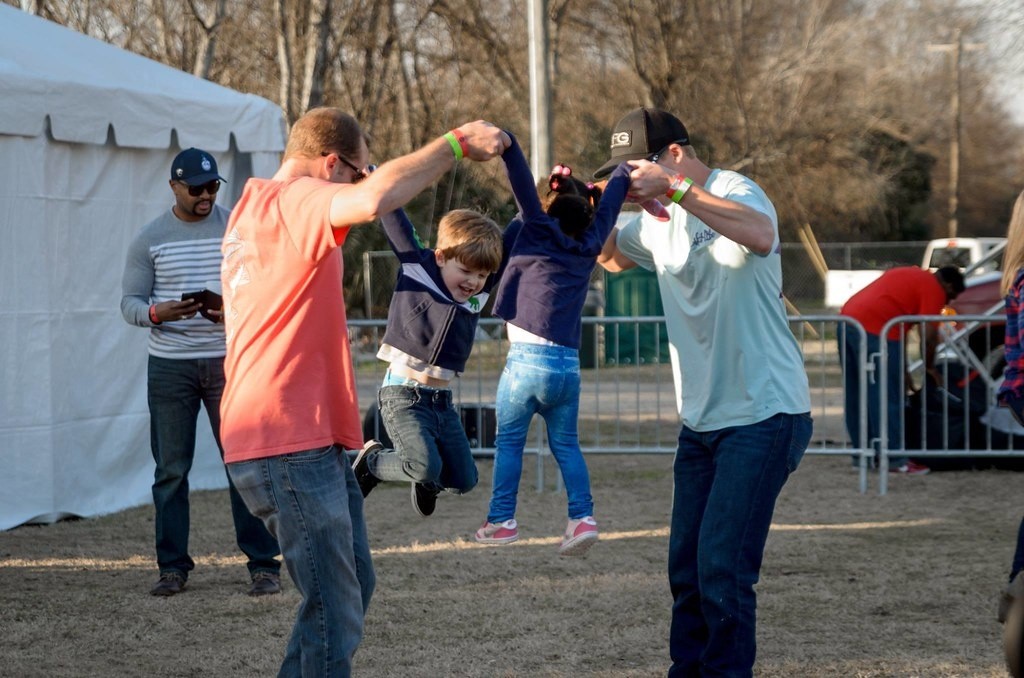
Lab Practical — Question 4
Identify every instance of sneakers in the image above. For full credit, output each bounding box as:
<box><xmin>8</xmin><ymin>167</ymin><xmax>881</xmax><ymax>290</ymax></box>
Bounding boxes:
<box><xmin>351</xmin><ymin>439</ymin><xmax>385</xmax><ymax>498</ymax></box>
<box><xmin>888</xmin><ymin>461</ymin><xmax>930</xmax><ymax>475</ymax></box>
<box><xmin>559</xmin><ymin>516</ymin><xmax>599</xmax><ymax>556</ymax></box>
<box><xmin>475</xmin><ymin>520</ymin><xmax>519</xmax><ymax>544</ymax></box>
<box><xmin>411</xmin><ymin>482</ymin><xmax>437</xmax><ymax>517</ymax></box>
<box><xmin>247</xmin><ymin>572</ymin><xmax>280</xmax><ymax>595</ymax></box>
<box><xmin>150</xmin><ymin>573</ymin><xmax>185</xmax><ymax>596</ymax></box>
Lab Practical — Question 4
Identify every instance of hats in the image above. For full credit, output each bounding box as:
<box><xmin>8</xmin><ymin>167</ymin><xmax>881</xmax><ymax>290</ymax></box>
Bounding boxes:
<box><xmin>171</xmin><ymin>148</ymin><xmax>227</xmax><ymax>185</ymax></box>
<box><xmin>593</xmin><ymin>107</ymin><xmax>693</xmax><ymax>178</ymax></box>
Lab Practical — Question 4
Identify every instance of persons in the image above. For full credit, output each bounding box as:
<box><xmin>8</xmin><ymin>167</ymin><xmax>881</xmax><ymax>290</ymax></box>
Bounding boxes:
<box><xmin>217</xmin><ymin>105</ymin><xmax>511</xmax><ymax>678</ymax></box>
<box><xmin>836</xmin><ymin>266</ymin><xmax>966</xmax><ymax>476</ymax></box>
<box><xmin>118</xmin><ymin>148</ymin><xmax>287</xmax><ymax>598</ymax></box>
<box><xmin>578</xmin><ymin>104</ymin><xmax>816</xmax><ymax>678</ymax></box>
<box><xmin>351</xmin><ymin>165</ymin><xmax>504</xmax><ymax>517</ymax></box>
<box><xmin>998</xmin><ymin>188</ymin><xmax>1024</xmax><ymax>678</ymax></box>
<box><xmin>476</xmin><ymin>128</ymin><xmax>631</xmax><ymax>554</ymax></box>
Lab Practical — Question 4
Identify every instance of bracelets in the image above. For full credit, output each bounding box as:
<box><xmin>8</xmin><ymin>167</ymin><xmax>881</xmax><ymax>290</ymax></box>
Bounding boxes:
<box><xmin>150</xmin><ymin>304</ymin><xmax>162</xmax><ymax>325</ymax></box>
<box><xmin>443</xmin><ymin>128</ymin><xmax>468</xmax><ymax>161</ymax></box>
<box><xmin>666</xmin><ymin>173</ymin><xmax>693</xmax><ymax>203</ymax></box>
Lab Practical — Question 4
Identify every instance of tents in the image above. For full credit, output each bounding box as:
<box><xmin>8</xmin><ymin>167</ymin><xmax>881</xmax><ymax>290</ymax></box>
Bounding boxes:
<box><xmin>0</xmin><ymin>0</ymin><xmax>290</xmax><ymax>535</ymax></box>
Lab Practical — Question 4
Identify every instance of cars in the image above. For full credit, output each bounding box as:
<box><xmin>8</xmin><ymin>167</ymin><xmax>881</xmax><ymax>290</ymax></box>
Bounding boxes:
<box><xmin>921</xmin><ymin>238</ymin><xmax>1010</xmax><ymax>285</ymax></box>
<box><xmin>938</xmin><ymin>270</ymin><xmax>1008</xmax><ymax>383</ymax></box>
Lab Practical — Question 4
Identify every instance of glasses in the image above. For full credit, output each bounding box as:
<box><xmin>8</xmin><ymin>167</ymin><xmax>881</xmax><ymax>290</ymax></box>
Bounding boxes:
<box><xmin>322</xmin><ymin>151</ymin><xmax>366</xmax><ymax>184</ymax></box>
<box><xmin>177</xmin><ymin>181</ymin><xmax>220</xmax><ymax>197</ymax></box>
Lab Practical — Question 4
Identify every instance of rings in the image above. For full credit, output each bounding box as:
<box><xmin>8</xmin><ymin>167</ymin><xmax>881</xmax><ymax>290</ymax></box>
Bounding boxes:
<box><xmin>181</xmin><ymin>315</ymin><xmax>188</xmax><ymax>320</ymax></box>
